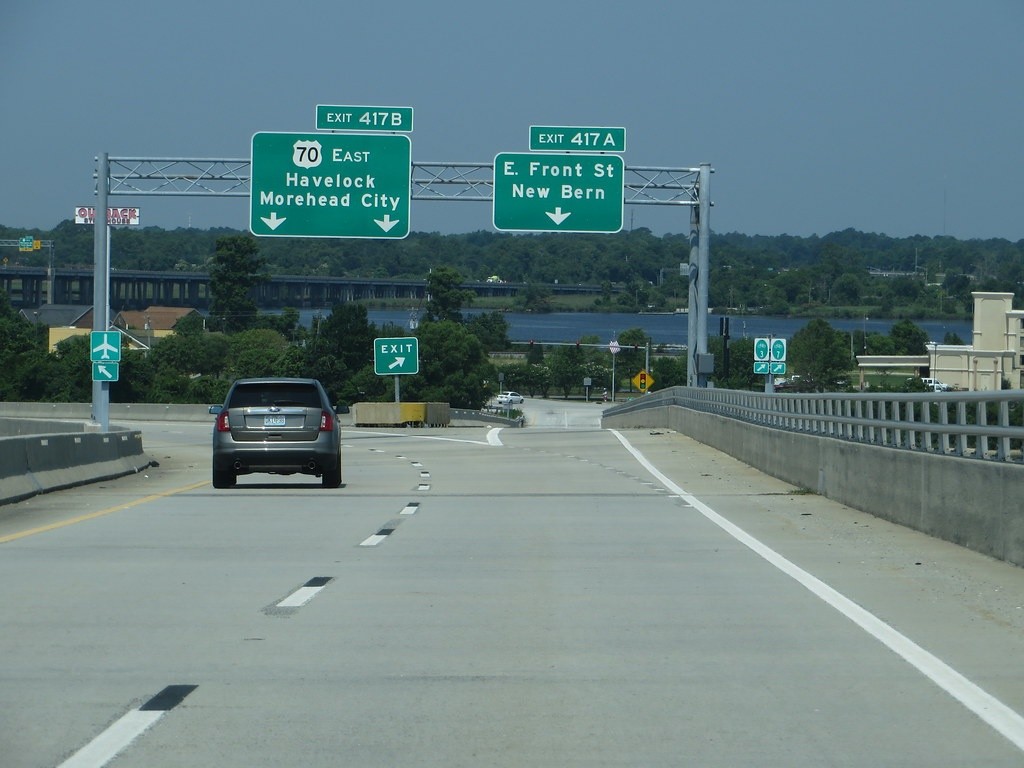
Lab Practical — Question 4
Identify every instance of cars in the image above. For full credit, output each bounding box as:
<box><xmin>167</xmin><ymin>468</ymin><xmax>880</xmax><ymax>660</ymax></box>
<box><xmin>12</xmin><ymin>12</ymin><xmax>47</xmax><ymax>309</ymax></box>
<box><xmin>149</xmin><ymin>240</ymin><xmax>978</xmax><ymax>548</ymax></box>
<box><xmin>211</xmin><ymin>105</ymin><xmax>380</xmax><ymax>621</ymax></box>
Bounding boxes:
<box><xmin>496</xmin><ymin>391</ymin><xmax>525</xmax><ymax>406</ymax></box>
<box><xmin>925</xmin><ymin>384</ymin><xmax>946</xmax><ymax>393</ymax></box>
<box><xmin>485</xmin><ymin>276</ymin><xmax>505</xmax><ymax>283</ymax></box>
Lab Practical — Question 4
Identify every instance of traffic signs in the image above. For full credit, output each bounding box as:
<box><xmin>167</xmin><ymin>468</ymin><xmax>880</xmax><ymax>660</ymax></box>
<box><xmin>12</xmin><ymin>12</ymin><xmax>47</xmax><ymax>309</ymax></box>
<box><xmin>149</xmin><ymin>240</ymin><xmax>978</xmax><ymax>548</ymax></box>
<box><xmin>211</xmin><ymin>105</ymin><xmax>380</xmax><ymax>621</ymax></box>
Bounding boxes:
<box><xmin>18</xmin><ymin>238</ymin><xmax>34</xmax><ymax>251</ymax></box>
<box><xmin>492</xmin><ymin>152</ymin><xmax>625</xmax><ymax>234</ymax></box>
<box><xmin>249</xmin><ymin>131</ymin><xmax>411</xmax><ymax>241</ymax></box>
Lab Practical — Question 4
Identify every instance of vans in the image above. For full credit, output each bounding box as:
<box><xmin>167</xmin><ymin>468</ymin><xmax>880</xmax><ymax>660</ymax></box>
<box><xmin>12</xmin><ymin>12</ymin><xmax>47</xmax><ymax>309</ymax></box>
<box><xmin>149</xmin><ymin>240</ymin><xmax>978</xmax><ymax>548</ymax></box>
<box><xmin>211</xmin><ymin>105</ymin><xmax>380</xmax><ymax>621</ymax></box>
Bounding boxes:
<box><xmin>791</xmin><ymin>375</ymin><xmax>813</xmax><ymax>383</ymax></box>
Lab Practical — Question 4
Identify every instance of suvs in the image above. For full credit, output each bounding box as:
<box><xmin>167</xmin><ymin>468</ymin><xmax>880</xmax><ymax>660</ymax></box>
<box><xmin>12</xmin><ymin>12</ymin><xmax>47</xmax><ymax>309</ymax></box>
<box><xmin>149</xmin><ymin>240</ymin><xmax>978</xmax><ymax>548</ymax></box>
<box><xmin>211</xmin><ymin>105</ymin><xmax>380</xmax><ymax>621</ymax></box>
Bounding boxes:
<box><xmin>922</xmin><ymin>377</ymin><xmax>947</xmax><ymax>390</ymax></box>
<box><xmin>207</xmin><ymin>376</ymin><xmax>343</xmax><ymax>490</ymax></box>
